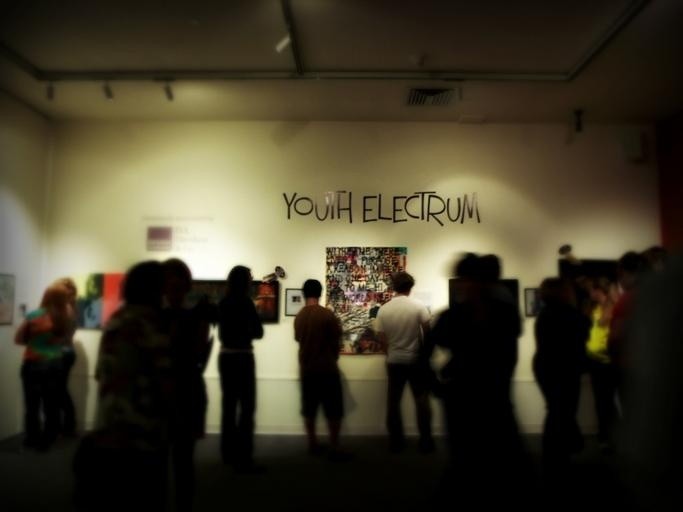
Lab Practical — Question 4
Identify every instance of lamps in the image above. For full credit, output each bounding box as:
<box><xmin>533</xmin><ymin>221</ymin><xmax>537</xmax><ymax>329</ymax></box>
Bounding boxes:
<box><xmin>45</xmin><ymin>78</ymin><xmax>56</xmax><ymax>98</ymax></box>
<box><xmin>101</xmin><ymin>80</ymin><xmax>114</xmax><ymax>99</ymax></box>
<box><xmin>163</xmin><ymin>81</ymin><xmax>174</xmax><ymax>99</ymax></box>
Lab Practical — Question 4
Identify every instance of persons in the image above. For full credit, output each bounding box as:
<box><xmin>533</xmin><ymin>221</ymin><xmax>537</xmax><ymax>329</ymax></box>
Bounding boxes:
<box><xmin>373</xmin><ymin>270</ymin><xmax>437</xmax><ymax>453</ymax></box>
<box><xmin>534</xmin><ymin>245</ymin><xmax>680</xmax><ymax>510</ymax></box>
<box><xmin>426</xmin><ymin>251</ymin><xmax>526</xmax><ymax>495</ymax></box>
<box><xmin>214</xmin><ymin>266</ymin><xmax>264</xmax><ymax>474</ymax></box>
<box><xmin>95</xmin><ymin>258</ymin><xmax>212</xmax><ymax>512</ymax></box>
<box><xmin>293</xmin><ymin>278</ymin><xmax>352</xmax><ymax>460</ymax></box>
<box><xmin>14</xmin><ymin>280</ymin><xmax>79</xmax><ymax>448</ymax></box>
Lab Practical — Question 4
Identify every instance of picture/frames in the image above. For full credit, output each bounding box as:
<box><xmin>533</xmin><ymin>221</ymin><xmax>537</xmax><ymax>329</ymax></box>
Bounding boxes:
<box><xmin>193</xmin><ymin>280</ymin><xmax>278</xmax><ymax>324</ymax></box>
<box><xmin>284</xmin><ymin>287</ymin><xmax>307</xmax><ymax>317</ymax></box>
<box><xmin>524</xmin><ymin>288</ymin><xmax>537</xmax><ymax>316</ymax></box>
